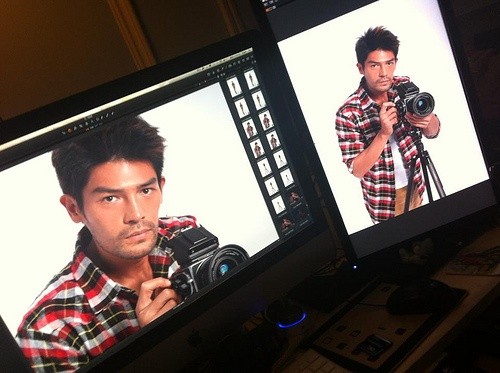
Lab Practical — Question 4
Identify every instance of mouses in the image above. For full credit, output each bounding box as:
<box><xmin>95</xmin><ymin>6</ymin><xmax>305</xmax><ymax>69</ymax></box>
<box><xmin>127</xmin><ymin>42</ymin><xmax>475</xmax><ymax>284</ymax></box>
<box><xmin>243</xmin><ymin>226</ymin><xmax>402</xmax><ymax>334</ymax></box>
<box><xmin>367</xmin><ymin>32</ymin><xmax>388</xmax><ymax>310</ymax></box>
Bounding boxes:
<box><xmin>385</xmin><ymin>278</ymin><xmax>456</xmax><ymax>314</ymax></box>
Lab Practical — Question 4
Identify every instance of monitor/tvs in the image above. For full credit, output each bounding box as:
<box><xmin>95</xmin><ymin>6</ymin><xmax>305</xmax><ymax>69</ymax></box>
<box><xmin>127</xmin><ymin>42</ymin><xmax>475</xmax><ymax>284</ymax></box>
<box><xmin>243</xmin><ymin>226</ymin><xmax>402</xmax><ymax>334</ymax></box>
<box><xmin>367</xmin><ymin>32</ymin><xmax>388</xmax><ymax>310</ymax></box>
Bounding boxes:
<box><xmin>0</xmin><ymin>55</ymin><xmax>339</xmax><ymax>373</ymax></box>
<box><xmin>253</xmin><ymin>0</ymin><xmax>500</xmax><ymax>262</ymax></box>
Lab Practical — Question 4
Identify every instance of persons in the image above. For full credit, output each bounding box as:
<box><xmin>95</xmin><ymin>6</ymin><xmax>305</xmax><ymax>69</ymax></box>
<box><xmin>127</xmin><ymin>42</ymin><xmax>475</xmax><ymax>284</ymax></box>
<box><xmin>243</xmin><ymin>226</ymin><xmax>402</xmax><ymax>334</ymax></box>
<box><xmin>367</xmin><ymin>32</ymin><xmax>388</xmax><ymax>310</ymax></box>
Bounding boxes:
<box><xmin>335</xmin><ymin>26</ymin><xmax>440</xmax><ymax>224</ymax></box>
<box><xmin>14</xmin><ymin>114</ymin><xmax>203</xmax><ymax>373</ymax></box>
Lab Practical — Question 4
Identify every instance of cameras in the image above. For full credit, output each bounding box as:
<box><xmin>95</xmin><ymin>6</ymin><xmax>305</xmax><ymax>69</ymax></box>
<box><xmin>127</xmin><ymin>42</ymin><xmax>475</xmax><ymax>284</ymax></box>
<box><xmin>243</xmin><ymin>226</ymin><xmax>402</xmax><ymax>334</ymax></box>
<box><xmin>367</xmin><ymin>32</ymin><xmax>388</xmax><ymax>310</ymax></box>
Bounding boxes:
<box><xmin>392</xmin><ymin>82</ymin><xmax>435</xmax><ymax>120</ymax></box>
<box><xmin>166</xmin><ymin>230</ymin><xmax>250</xmax><ymax>298</ymax></box>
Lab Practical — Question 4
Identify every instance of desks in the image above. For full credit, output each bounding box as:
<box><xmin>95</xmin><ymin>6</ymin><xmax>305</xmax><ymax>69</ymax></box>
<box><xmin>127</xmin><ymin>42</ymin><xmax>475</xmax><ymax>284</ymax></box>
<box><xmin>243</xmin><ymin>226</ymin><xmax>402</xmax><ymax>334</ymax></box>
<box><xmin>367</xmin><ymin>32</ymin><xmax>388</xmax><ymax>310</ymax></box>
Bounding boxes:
<box><xmin>276</xmin><ymin>218</ymin><xmax>500</xmax><ymax>373</ymax></box>
